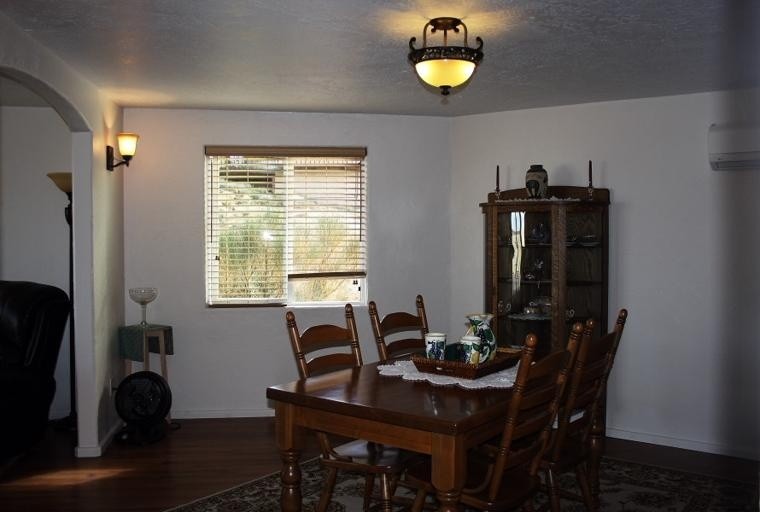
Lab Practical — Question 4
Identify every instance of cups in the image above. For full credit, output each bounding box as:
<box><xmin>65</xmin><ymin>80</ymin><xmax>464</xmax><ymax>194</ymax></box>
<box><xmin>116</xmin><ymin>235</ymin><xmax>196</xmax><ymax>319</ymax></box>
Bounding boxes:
<box><xmin>424</xmin><ymin>331</ymin><xmax>449</xmax><ymax>360</ymax></box>
<box><xmin>456</xmin><ymin>395</ymin><xmax>498</xmax><ymax>414</ymax></box>
<box><xmin>423</xmin><ymin>388</ymin><xmax>446</xmax><ymax>417</ymax></box>
<box><xmin>457</xmin><ymin>335</ymin><xmax>490</xmax><ymax>364</ymax></box>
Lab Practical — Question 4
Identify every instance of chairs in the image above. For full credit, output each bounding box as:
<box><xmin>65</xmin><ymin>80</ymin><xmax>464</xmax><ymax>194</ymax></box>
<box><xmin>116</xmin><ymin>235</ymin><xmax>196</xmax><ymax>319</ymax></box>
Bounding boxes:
<box><xmin>0</xmin><ymin>279</ymin><xmax>69</xmax><ymax>480</ymax></box>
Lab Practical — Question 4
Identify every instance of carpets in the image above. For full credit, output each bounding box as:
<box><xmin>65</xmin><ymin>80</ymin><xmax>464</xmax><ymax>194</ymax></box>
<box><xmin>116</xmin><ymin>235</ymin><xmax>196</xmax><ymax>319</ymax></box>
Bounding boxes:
<box><xmin>147</xmin><ymin>436</ymin><xmax>759</xmax><ymax>512</ymax></box>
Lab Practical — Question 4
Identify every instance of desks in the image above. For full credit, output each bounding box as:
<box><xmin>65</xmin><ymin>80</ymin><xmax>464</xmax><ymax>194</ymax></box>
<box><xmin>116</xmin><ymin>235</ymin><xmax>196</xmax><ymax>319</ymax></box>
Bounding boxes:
<box><xmin>115</xmin><ymin>325</ymin><xmax>173</xmax><ymax>439</ymax></box>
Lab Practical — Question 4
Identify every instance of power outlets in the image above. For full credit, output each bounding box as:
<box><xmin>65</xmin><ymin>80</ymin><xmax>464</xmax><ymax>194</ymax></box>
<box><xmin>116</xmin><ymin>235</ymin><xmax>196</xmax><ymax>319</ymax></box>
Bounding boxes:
<box><xmin>110</xmin><ymin>378</ymin><xmax>115</xmax><ymax>397</ymax></box>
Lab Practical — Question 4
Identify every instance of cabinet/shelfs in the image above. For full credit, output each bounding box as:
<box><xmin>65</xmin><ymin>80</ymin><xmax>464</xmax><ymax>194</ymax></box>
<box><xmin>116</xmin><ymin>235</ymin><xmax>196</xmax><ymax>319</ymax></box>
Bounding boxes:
<box><xmin>480</xmin><ymin>186</ymin><xmax>607</xmax><ymax>445</ymax></box>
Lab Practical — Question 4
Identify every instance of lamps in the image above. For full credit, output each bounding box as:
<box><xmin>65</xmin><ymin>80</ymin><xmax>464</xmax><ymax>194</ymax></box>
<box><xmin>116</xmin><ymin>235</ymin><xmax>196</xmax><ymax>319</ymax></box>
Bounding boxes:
<box><xmin>406</xmin><ymin>17</ymin><xmax>487</xmax><ymax>99</ymax></box>
<box><xmin>47</xmin><ymin>171</ymin><xmax>77</xmax><ymax>445</ymax></box>
<box><xmin>104</xmin><ymin>132</ymin><xmax>138</xmax><ymax>170</ymax></box>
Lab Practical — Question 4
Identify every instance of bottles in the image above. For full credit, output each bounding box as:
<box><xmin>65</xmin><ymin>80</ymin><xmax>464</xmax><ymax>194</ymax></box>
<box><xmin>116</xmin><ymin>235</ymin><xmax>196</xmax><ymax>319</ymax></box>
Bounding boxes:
<box><xmin>526</xmin><ymin>164</ymin><xmax>548</xmax><ymax>200</ymax></box>
<box><xmin>463</xmin><ymin>312</ymin><xmax>498</xmax><ymax>361</ymax></box>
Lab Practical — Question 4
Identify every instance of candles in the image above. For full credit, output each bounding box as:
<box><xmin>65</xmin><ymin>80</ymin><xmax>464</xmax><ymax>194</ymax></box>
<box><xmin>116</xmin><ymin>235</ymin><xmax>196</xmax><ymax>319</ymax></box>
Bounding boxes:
<box><xmin>588</xmin><ymin>160</ymin><xmax>593</xmax><ymax>185</ymax></box>
<box><xmin>495</xmin><ymin>165</ymin><xmax>500</xmax><ymax>192</ymax></box>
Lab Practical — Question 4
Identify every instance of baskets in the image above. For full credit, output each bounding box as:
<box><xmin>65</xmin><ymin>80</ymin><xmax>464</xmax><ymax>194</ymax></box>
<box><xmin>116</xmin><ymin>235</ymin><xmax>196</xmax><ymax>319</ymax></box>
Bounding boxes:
<box><xmin>411</xmin><ymin>343</ymin><xmax>522</xmax><ymax>380</ymax></box>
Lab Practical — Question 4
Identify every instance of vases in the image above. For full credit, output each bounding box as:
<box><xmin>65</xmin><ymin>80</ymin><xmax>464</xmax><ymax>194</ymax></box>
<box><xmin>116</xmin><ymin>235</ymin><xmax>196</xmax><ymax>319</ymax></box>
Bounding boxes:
<box><xmin>524</xmin><ymin>164</ymin><xmax>550</xmax><ymax>198</ymax></box>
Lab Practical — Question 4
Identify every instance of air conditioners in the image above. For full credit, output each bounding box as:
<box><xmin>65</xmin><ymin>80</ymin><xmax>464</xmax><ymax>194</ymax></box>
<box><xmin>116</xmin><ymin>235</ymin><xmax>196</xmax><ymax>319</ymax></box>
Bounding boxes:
<box><xmin>708</xmin><ymin>123</ymin><xmax>760</xmax><ymax>172</ymax></box>
